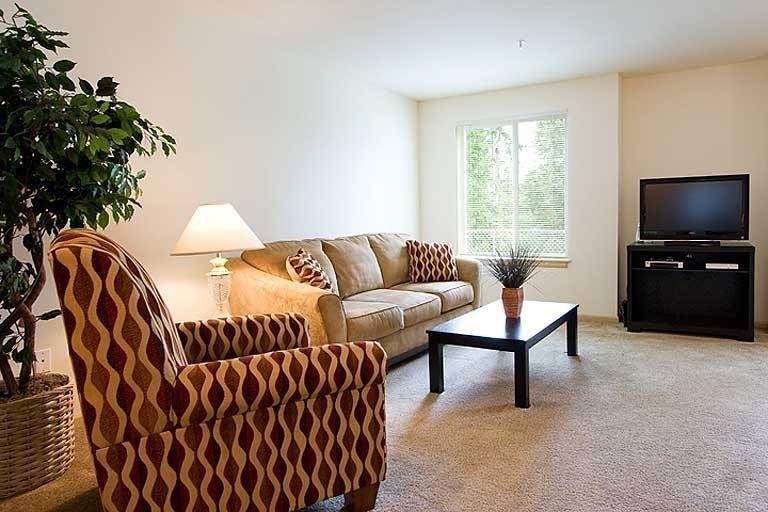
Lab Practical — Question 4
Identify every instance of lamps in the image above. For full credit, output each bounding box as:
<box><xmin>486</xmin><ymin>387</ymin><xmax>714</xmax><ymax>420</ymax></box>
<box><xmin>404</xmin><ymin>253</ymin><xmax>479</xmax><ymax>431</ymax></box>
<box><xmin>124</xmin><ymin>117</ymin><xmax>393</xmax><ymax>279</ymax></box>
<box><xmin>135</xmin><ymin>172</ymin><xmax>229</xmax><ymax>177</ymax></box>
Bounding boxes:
<box><xmin>169</xmin><ymin>202</ymin><xmax>267</xmax><ymax>319</ymax></box>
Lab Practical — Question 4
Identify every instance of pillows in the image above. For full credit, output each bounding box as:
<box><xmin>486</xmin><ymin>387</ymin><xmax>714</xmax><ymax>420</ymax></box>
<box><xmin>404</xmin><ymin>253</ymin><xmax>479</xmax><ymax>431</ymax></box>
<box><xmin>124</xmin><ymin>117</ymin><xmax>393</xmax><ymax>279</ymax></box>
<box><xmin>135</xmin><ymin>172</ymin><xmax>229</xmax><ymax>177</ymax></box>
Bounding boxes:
<box><xmin>406</xmin><ymin>238</ymin><xmax>459</xmax><ymax>282</ymax></box>
<box><xmin>284</xmin><ymin>247</ymin><xmax>336</xmax><ymax>295</ymax></box>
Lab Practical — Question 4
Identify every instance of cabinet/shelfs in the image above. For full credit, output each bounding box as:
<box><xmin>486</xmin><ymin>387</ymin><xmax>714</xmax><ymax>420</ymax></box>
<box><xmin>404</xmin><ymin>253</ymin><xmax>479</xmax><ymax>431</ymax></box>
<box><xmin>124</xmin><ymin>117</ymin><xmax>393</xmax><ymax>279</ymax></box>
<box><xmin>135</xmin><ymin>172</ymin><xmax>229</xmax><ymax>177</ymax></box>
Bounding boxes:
<box><xmin>622</xmin><ymin>240</ymin><xmax>753</xmax><ymax>342</ymax></box>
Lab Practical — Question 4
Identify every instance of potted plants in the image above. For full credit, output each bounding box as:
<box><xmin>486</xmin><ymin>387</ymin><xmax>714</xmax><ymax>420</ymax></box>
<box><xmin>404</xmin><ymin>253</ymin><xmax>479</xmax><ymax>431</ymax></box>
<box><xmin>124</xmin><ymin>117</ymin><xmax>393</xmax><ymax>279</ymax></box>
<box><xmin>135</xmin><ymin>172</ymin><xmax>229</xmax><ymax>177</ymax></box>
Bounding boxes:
<box><xmin>485</xmin><ymin>237</ymin><xmax>542</xmax><ymax>322</ymax></box>
<box><xmin>0</xmin><ymin>3</ymin><xmax>171</xmax><ymax>498</ymax></box>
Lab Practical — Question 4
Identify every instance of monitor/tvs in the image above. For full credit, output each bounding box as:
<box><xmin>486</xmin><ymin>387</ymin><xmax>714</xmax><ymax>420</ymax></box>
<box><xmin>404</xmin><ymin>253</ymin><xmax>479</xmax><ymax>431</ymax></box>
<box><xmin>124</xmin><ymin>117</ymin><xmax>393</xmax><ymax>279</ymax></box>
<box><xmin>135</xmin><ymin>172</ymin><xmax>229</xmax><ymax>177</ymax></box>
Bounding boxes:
<box><xmin>640</xmin><ymin>174</ymin><xmax>749</xmax><ymax>246</ymax></box>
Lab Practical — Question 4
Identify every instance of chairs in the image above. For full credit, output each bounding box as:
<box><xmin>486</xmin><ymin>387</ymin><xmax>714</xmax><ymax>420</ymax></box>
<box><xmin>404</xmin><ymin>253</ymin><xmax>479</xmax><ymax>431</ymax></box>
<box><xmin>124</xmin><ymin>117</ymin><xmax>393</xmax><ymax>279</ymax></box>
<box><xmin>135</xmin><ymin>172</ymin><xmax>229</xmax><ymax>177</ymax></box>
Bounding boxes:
<box><xmin>51</xmin><ymin>228</ymin><xmax>384</xmax><ymax>512</ymax></box>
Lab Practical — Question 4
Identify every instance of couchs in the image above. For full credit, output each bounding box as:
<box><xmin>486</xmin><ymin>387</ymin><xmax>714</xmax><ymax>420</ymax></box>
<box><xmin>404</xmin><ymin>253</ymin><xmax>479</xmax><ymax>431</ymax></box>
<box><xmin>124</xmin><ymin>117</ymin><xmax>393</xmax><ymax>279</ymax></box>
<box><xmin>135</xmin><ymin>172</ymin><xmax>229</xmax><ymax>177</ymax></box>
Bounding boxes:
<box><xmin>222</xmin><ymin>232</ymin><xmax>479</xmax><ymax>377</ymax></box>
